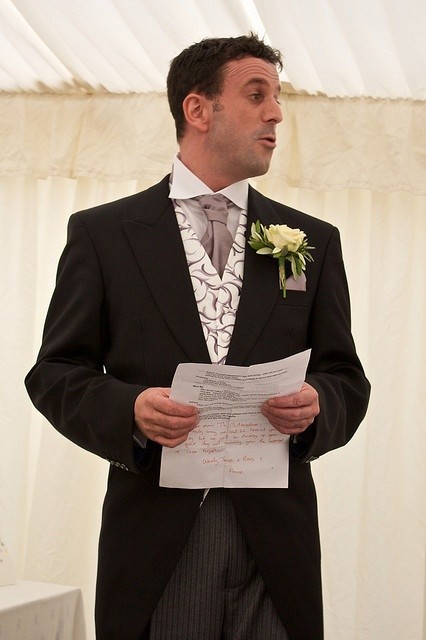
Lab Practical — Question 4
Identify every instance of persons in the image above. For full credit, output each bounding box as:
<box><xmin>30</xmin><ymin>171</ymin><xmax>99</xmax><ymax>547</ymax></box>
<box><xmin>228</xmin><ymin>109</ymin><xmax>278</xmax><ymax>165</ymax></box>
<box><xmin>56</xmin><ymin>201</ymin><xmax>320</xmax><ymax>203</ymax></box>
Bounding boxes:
<box><xmin>24</xmin><ymin>30</ymin><xmax>371</xmax><ymax>640</ymax></box>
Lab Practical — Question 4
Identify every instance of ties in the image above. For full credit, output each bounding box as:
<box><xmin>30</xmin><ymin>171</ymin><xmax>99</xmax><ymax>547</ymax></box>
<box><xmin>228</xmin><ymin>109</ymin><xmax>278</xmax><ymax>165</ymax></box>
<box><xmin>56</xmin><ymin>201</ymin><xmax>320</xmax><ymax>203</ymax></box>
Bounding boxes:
<box><xmin>189</xmin><ymin>193</ymin><xmax>235</xmax><ymax>282</ymax></box>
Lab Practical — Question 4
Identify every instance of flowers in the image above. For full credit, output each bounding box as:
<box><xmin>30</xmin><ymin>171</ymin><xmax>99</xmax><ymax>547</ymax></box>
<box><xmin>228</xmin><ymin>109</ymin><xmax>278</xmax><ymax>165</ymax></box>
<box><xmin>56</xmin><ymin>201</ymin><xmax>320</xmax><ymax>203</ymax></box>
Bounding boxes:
<box><xmin>246</xmin><ymin>220</ymin><xmax>317</xmax><ymax>300</ymax></box>
